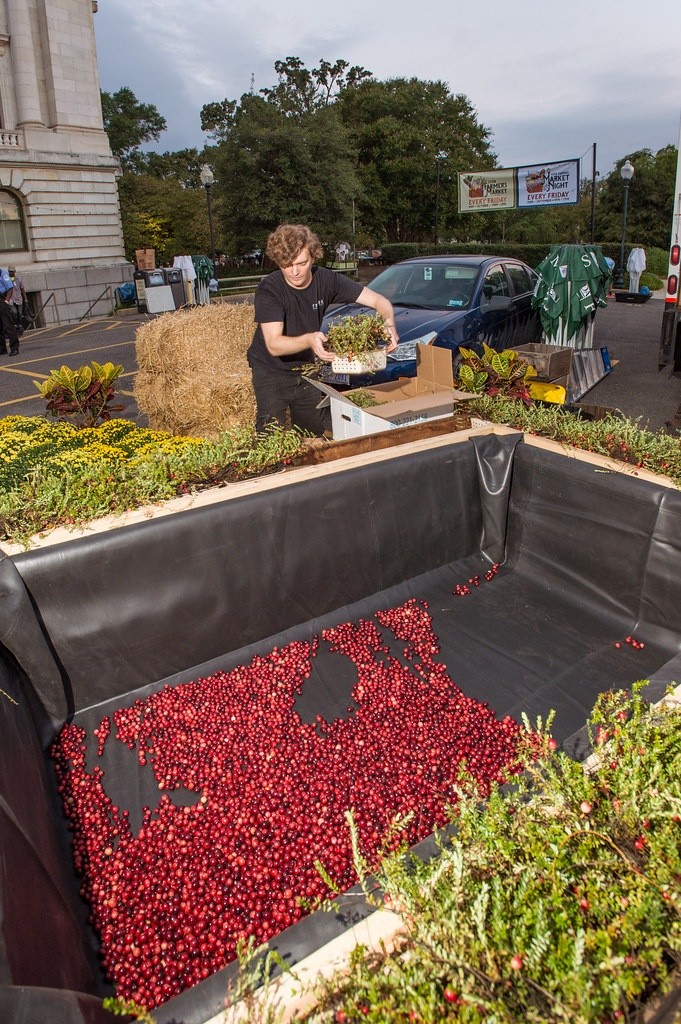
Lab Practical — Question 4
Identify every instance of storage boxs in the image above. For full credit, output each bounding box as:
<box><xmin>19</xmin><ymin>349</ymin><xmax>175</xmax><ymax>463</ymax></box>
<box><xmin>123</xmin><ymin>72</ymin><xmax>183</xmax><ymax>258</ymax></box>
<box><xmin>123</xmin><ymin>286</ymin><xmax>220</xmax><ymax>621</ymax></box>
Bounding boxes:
<box><xmin>135</xmin><ymin>249</ymin><xmax>156</xmax><ymax>271</ymax></box>
<box><xmin>546</xmin><ymin>375</ymin><xmax>569</xmax><ymax>396</ymax></box>
<box><xmin>301</xmin><ymin>343</ymin><xmax>483</xmax><ymax>442</ymax></box>
<box><xmin>506</xmin><ymin>343</ymin><xmax>573</xmax><ymax>382</ymax></box>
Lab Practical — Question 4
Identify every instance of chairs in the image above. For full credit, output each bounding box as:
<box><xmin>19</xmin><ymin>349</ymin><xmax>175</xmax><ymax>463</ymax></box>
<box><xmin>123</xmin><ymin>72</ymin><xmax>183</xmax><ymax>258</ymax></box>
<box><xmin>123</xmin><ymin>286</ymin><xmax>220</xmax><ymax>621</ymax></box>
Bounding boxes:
<box><xmin>480</xmin><ymin>287</ymin><xmax>494</xmax><ymax>302</ymax></box>
<box><xmin>525</xmin><ymin>383</ymin><xmax>566</xmax><ymax>414</ymax></box>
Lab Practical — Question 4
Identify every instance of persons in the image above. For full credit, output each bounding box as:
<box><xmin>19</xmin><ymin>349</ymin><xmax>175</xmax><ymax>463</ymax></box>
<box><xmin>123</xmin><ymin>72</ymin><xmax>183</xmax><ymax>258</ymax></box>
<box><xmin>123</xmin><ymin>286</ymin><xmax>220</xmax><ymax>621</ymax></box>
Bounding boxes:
<box><xmin>246</xmin><ymin>222</ymin><xmax>400</xmax><ymax>444</ymax></box>
<box><xmin>0</xmin><ymin>265</ymin><xmax>29</xmax><ymax>356</ymax></box>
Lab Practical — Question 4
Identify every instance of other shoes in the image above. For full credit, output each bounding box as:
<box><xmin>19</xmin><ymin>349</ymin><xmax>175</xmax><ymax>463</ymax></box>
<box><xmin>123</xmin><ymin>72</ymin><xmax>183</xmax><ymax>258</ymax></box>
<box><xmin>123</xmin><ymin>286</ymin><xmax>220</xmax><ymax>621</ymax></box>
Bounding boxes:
<box><xmin>9</xmin><ymin>350</ymin><xmax>19</xmax><ymax>356</ymax></box>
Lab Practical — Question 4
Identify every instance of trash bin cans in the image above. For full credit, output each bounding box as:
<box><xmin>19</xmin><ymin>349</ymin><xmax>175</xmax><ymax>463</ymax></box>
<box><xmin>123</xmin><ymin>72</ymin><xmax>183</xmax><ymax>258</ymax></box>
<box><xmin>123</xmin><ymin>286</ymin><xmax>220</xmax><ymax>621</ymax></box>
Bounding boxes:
<box><xmin>133</xmin><ymin>268</ymin><xmax>165</xmax><ymax>314</ymax></box>
<box><xmin>159</xmin><ymin>267</ymin><xmax>185</xmax><ymax>309</ymax></box>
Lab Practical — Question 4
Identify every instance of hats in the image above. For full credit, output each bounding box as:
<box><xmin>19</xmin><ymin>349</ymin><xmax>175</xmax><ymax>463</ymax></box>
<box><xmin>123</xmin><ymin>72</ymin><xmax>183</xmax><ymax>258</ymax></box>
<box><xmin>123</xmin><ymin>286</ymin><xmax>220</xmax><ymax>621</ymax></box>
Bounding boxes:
<box><xmin>8</xmin><ymin>266</ymin><xmax>15</xmax><ymax>272</ymax></box>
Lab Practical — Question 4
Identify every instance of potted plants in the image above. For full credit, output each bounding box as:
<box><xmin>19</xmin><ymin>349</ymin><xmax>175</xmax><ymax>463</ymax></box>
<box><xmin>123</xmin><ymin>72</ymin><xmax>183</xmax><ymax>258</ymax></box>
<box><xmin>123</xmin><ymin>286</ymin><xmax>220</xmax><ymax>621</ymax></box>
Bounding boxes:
<box><xmin>292</xmin><ymin>309</ymin><xmax>393</xmax><ymax>388</ymax></box>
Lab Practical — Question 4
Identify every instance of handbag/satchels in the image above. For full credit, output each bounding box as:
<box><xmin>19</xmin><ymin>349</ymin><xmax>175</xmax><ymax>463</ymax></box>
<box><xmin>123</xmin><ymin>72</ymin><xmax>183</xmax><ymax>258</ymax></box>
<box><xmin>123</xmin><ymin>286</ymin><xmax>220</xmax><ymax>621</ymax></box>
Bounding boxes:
<box><xmin>22</xmin><ymin>303</ymin><xmax>35</xmax><ymax>323</ymax></box>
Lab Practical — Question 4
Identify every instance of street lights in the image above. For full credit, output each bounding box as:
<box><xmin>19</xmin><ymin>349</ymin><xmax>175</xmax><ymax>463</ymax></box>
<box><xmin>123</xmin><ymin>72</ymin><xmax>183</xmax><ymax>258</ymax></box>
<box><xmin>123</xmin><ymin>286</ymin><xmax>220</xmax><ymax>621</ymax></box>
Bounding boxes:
<box><xmin>200</xmin><ymin>163</ymin><xmax>216</xmax><ymax>276</ymax></box>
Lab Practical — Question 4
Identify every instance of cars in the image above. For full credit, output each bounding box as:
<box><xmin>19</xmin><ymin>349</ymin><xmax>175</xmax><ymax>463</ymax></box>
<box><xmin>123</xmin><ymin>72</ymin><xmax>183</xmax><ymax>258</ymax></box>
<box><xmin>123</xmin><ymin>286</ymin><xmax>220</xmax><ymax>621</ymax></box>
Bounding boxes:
<box><xmin>309</xmin><ymin>254</ymin><xmax>543</xmax><ymax>388</ymax></box>
<box><xmin>357</xmin><ymin>251</ymin><xmax>375</xmax><ymax>267</ymax></box>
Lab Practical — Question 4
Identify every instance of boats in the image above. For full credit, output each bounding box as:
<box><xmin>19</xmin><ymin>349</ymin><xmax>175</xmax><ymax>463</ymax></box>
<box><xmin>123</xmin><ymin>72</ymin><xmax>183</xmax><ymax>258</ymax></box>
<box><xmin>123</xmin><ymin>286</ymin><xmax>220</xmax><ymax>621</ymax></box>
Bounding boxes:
<box><xmin>613</xmin><ymin>159</ymin><xmax>635</xmax><ymax>288</ymax></box>
